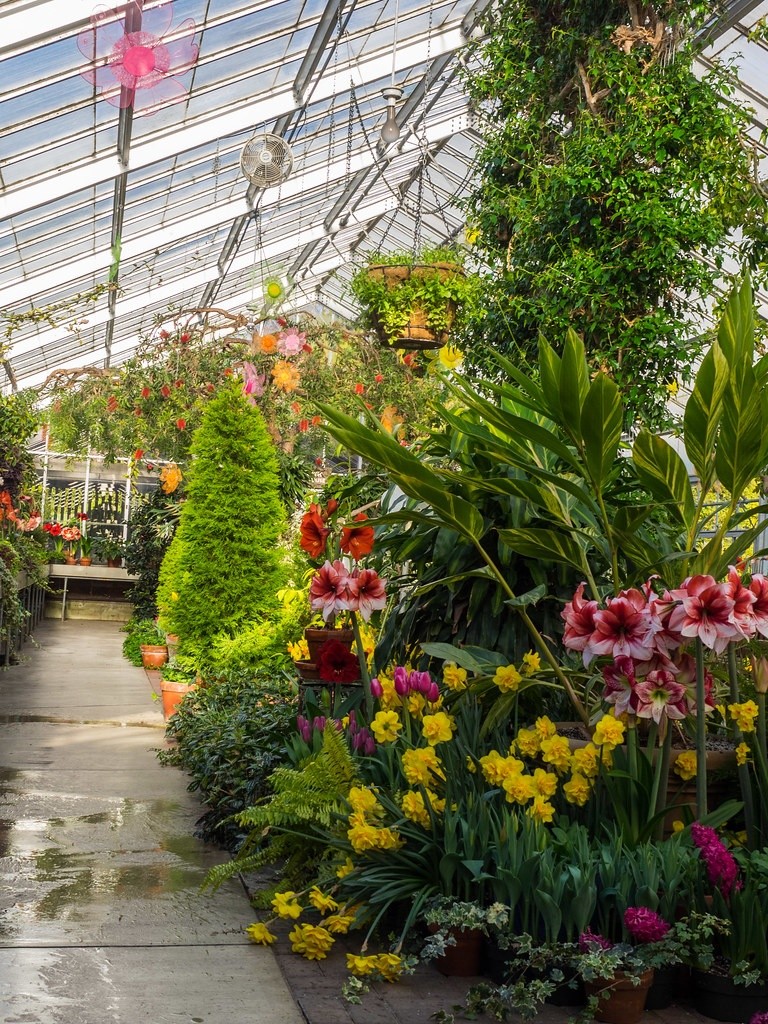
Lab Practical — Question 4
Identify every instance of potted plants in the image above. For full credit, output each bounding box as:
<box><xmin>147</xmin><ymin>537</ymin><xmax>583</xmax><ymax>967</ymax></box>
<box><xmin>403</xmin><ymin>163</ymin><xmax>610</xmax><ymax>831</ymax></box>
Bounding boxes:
<box><xmin>680</xmin><ymin>944</ymin><xmax>768</xmax><ymax>1022</ymax></box>
<box><xmin>160</xmin><ymin>668</ymin><xmax>196</xmax><ymax>720</ymax></box>
<box><xmin>121</xmin><ymin>624</ymin><xmax>167</xmax><ymax>669</ymax></box>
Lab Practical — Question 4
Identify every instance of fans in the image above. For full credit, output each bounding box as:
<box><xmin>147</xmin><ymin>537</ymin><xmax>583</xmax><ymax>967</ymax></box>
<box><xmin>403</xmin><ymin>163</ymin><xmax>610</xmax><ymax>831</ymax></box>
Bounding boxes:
<box><xmin>239</xmin><ymin>135</ymin><xmax>294</xmax><ymax>189</ymax></box>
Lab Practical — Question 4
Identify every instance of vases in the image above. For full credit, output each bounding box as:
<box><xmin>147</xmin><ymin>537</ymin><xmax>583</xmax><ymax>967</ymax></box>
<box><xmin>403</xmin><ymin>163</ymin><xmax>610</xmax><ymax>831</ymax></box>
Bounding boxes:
<box><xmin>426</xmin><ymin>921</ymin><xmax>482</xmax><ymax>974</ymax></box>
<box><xmin>645</xmin><ymin>964</ymin><xmax>681</xmax><ymax>1010</ymax></box>
<box><xmin>361</xmin><ymin>262</ymin><xmax>465</xmax><ymax>350</ymax></box>
<box><xmin>51</xmin><ymin>558</ymin><xmax>64</xmax><ymax>564</ymax></box>
<box><xmin>296</xmin><ymin>660</ymin><xmax>368</xmax><ymax>680</ymax></box>
<box><xmin>80</xmin><ymin>557</ymin><xmax>92</xmax><ymax>566</ymax></box>
<box><xmin>63</xmin><ymin>550</ymin><xmax>75</xmax><ymax>557</ymax></box>
<box><xmin>528</xmin><ymin>967</ymin><xmax>589</xmax><ymax>1006</ymax></box>
<box><xmin>166</xmin><ymin>635</ymin><xmax>178</xmax><ymax>662</ymax></box>
<box><xmin>66</xmin><ymin>557</ymin><xmax>77</xmax><ymax>564</ymax></box>
<box><xmin>597</xmin><ymin>970</ymin><xmax>654</xmax><ymax>1024</ymax></box>
<box><xmin>305</xmin><ymin>628</ymin><xmax>354</xmax><ymax>662</ymax></box>
<box><xmin>108</xmin><ymin>557</ymin><xmax>120</xmax><ymax>567</ymax></box>
<box><xmin>550</xmin><ymin>721</ymin><xmax>740</xmax><ymax>838</ymax></box>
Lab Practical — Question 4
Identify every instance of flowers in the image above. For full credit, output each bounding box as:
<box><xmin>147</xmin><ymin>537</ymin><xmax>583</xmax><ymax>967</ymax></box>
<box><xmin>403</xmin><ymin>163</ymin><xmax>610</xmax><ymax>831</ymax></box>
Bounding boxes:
<box><xmin>77</xmin><ymin>0</ymin><xmax>199</xmax><ymax>119</ymax></box>
<box><xmin>252</xmin><ymin>330</ymin><xmax>279</xmax><ymax>355</ymax></box>
<box><xmin>271</xmin><ymin>360</ymin><xmax>300</xmax><ymax>393</ymax></box>
<box><xmin>160</xmin><ymin>462</ymin><xmax>182</xmax><ymax>495</ymax></box>
<box><xmin>278</xmin><ymin>327</ymin><xmax>307</xmax><ymax>356</ymax></box>
<box><xmin>238</xmin><ymin>361</ymin><xmax>267</xmax><ymax>405</ymax></box>
<box><xmin>263</xmin><ymin>276</ymin><xmax>285</xmax><ymax>304</ymax></box>
<box><xmin>0</xmin><ymin>229</ymin><xmax>768</xmax><ymax>1024</ymax></box>
